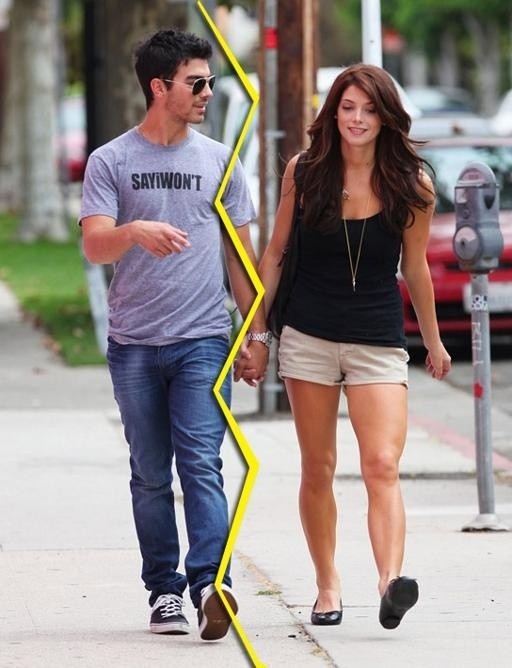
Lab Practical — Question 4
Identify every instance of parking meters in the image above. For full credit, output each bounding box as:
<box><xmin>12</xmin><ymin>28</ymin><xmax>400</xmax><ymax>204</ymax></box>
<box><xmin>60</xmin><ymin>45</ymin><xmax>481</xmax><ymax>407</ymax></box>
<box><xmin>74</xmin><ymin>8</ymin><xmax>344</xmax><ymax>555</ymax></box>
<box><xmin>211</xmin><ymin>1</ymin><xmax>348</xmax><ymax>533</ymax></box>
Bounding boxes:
<box><xmin>453</xmin><ymin>162</ymin><xmax>506</xmax><ymax>530</ymax></box>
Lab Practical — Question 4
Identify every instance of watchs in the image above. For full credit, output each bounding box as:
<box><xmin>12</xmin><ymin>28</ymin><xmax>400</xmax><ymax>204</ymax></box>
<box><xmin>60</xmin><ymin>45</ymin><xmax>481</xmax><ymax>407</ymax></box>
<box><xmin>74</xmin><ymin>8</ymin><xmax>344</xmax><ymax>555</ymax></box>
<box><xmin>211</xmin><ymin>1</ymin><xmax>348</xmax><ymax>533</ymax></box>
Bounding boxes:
<box><xmin>245</xmin><ymin>329</ymin><xmax>274</xmax><ymax>348</ymax></box>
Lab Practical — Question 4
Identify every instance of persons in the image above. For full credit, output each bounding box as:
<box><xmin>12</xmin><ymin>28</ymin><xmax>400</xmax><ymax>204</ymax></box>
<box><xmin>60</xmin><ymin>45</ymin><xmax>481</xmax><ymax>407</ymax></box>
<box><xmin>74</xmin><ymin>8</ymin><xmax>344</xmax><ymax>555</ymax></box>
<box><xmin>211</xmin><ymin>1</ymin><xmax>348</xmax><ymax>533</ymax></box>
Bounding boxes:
<box><xmin>77</xmin><ymin>28</ymin><xmax>273</xmax><ymax>643</ymax></box>
<box><xmin>232</xmin><ymin>61</ymin><xmax>455</xmax><ymax>632</ymax></box>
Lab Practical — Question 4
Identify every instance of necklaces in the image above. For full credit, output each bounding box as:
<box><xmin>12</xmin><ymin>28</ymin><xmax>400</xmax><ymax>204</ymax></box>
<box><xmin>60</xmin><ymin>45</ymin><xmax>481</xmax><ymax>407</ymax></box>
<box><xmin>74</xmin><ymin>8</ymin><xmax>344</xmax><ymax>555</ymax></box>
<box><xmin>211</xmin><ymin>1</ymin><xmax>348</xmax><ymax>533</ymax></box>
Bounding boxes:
<box><xmin>343</xmin><ymin>188</ymin><xmax>376</xmax><ymax>295</ymax></box>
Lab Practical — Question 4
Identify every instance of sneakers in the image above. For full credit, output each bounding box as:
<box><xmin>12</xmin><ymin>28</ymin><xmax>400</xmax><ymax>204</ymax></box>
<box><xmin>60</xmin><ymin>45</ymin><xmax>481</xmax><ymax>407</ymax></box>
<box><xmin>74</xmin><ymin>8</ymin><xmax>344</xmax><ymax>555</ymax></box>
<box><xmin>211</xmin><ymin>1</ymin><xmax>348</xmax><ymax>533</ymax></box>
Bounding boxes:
<box><xmin>194</xmin><ymin>574</ymin><xmax>239</xmax><ymax>642</ymax></box>
<box><xmin>149</xmin><ymin>594</ymin><xmax>191</xmax><ymax>636</ymax></box>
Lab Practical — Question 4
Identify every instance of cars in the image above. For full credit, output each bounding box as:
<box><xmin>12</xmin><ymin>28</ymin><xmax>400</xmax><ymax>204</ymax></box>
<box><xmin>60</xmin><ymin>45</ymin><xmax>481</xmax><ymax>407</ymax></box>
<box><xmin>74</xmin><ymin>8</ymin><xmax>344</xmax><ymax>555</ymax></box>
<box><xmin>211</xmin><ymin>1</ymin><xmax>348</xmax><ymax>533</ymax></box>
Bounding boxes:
<box><xmin>53</xmin><ymin>96</ymin><xmax>86</xmax><ymax>181</ymax></box>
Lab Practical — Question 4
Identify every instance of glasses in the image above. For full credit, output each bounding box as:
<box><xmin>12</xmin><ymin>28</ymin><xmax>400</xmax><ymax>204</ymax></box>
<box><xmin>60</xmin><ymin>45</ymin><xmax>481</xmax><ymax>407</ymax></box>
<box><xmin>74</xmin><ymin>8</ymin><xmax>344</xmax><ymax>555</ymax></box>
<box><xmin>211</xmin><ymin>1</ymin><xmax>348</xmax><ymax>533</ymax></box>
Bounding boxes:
<box><xmin>159</xmin><ymin>72</ymin><xmax>219</xmax><ymax>96</ymax></box>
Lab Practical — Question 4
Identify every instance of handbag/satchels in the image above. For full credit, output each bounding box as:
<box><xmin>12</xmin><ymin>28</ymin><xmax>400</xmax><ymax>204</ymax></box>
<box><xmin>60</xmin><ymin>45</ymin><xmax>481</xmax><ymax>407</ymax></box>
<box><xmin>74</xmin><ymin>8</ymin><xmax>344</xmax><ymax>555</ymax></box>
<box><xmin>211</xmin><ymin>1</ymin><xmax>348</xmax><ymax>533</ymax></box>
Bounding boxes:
<box><xmin>263</xmin><ymin>148</ymin><xmax>311</xmax><ymax>341</ymax></box>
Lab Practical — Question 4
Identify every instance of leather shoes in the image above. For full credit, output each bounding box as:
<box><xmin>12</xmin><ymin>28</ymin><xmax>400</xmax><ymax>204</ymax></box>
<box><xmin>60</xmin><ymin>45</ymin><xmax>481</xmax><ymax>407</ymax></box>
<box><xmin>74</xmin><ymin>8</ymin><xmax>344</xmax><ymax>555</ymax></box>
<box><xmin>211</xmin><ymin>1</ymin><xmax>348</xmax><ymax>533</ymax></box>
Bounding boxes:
<box><xmin>377</xmin><ymin>574</ymin><xmax>420</xmax><ymax>630</ymax></box>
<box><xmin>309</xmin><ymin>592</ymin><xmax>343</xmax><ymax>628</ymax></box>
